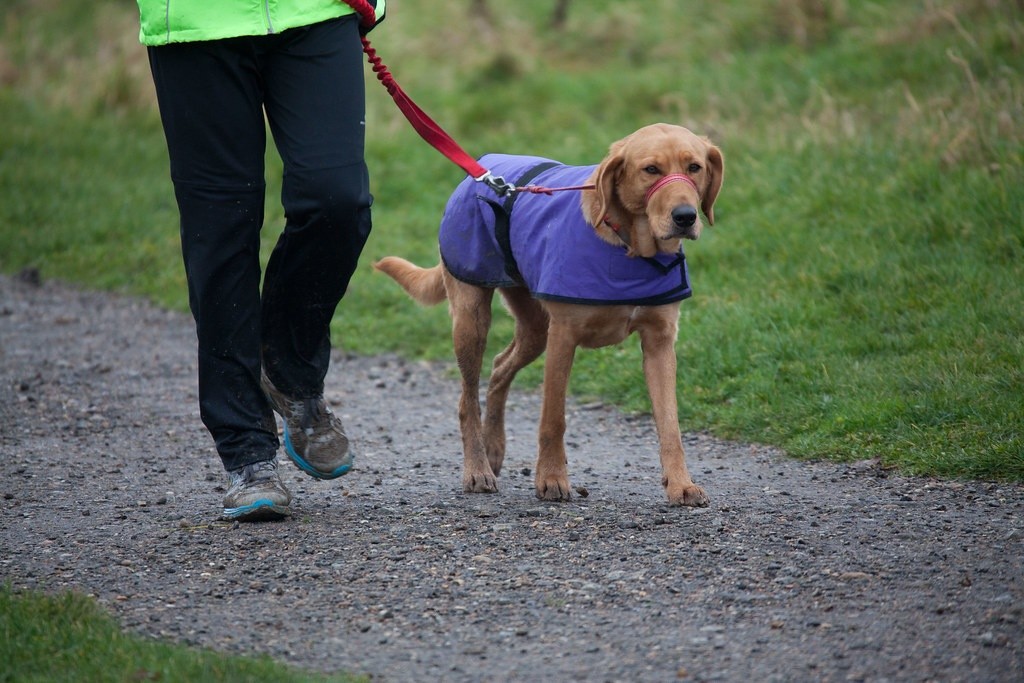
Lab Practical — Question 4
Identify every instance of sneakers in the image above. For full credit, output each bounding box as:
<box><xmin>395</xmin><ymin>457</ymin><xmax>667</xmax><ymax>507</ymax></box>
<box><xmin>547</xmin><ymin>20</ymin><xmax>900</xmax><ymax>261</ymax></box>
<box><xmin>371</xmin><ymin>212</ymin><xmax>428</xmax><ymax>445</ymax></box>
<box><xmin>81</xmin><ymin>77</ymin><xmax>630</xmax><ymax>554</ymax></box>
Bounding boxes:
<box><xmin>260</xmin><ymin>367</ymin><xmax>352</xmax><ymax>479</ymax></box>
<box><xmin>223</xmin><ymin>455</ymin><xmax>291</xmax><ymax>519</ymax></box>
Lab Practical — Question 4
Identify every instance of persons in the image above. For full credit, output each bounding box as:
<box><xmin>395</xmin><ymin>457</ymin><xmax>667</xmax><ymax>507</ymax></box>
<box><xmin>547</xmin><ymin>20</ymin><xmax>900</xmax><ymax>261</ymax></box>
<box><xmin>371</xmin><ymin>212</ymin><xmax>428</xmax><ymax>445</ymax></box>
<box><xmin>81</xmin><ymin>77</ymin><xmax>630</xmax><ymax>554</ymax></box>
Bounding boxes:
<box><xmin>136</xmin><ymin>0</ymin><xmax>387</xmax><ymax>521</ymax></box>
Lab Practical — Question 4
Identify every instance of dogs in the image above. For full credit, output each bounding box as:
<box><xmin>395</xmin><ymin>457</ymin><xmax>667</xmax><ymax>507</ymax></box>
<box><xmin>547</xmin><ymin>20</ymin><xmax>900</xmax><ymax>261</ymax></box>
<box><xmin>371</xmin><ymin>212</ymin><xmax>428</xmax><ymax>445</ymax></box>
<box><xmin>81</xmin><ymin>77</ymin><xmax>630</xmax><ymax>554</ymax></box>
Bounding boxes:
<box><xmin>375</xmin><ymin>122</ymin><xmax>724</xmax><ymax>507</ymax></box>
